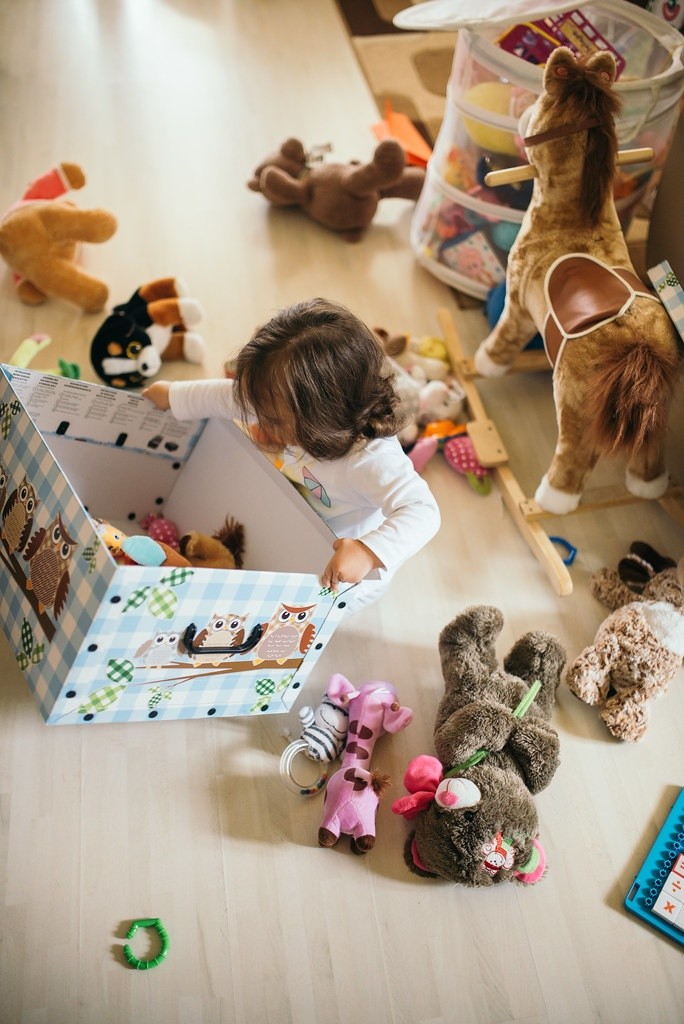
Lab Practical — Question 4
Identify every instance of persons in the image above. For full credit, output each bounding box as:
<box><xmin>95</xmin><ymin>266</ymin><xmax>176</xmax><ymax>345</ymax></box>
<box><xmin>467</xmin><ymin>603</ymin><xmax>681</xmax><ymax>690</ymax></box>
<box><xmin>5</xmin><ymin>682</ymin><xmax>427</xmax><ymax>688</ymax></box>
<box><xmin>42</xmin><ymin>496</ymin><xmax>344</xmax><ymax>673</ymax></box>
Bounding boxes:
<box><xmin>141</xmin><ymin>297</ymin><xmax>442</xmax><ymax>621</ymax></box>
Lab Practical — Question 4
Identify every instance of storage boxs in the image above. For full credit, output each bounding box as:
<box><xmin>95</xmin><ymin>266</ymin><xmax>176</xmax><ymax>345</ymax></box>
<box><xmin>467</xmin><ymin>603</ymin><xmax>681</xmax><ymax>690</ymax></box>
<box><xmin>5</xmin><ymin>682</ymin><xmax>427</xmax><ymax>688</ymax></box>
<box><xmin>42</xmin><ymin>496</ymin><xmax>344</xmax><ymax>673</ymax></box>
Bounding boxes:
<box><xmin>0</xmin><ymin>362</ymin><xmax>354</xmax><ymax>725</ymax></box>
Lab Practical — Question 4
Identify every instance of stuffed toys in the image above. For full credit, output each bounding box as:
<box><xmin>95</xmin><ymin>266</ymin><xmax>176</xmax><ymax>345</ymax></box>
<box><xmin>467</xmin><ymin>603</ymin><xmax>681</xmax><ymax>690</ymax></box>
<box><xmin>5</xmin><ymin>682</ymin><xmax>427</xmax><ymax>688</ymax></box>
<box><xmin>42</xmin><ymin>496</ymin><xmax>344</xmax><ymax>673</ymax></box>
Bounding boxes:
<box><xmin>246</xmin><ymin>138</ymin><xmax>428</xmax><ymax>243</ymax></box>
<box><xmin>317</xmin><ymin>674</ymin><xmax>414</xmax><ymax>853</ymax></box>
<box><xmin>393</xmin><ymin>602</ymin><xmax>565</xmax><ymax>889</ymax></box>
<box><xmin>89</xmin><ymin>277</ymin><xmax>207</xmax><ymax>388</ymax></box>
<box><xmin>566</xmin><ymin>559</ymin><xmax>684</xmax><ymax>742</ymax></box>
<box><xmin>375</xmin><ymin>327</ymin><xmax>496</xmax><ymax>497</ymax></box>
<box><xmin>286</xmin><ymin>692</ymin><xmax>351</xmax><ymax>763</ymax></box>
<box><xmin>0</xmin><ymin>162</ymin><xmax>118</xmax><ymax>314</ymax></box>
<box><xmin>472</xmin><ymin>44</ymin><xmax>678</xmax><ymax>515</ymax></box>
<box><xmin>88</xmin><ymin>512</ymin><xmax>247</xmax><ymax>570</ymax></box>
